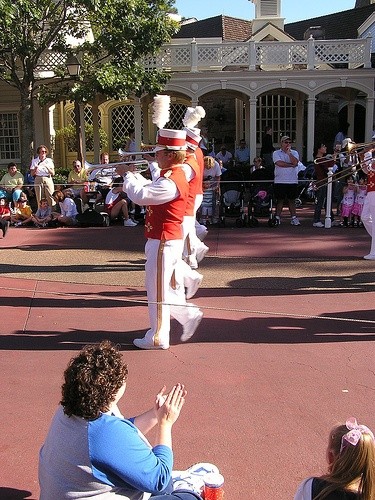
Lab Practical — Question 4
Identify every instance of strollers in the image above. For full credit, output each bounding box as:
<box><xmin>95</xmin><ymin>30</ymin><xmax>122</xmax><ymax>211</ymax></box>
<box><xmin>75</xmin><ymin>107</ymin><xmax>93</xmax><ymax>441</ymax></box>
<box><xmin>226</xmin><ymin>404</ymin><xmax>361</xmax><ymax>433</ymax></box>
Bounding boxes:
<box><xmin>295</xmin><ymin>164</ymin><xmax>316</xmax><ymax>208</ymax></box>
<box><xmin>218</xmin><ymin>168</ymin><xmax>246</xmax><ymax>226</ymax></box>
<box><xmin>246</xmin><ymin>168</ymin><xmax>276</xmax><ymax>227</ymax></box>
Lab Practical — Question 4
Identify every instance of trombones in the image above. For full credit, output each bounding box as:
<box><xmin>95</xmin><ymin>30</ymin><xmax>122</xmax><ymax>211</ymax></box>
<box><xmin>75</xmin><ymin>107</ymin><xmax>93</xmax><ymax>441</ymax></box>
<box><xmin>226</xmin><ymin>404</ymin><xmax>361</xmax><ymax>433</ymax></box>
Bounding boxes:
<box><xmin>314</xmin><ymin>151</ymin><xmax>375</xmax><ymax>191</ymax></box>
<box><xmin>314</xmin><ymin>140</ymin><xmax>375</xmax><ymax>165</ymax></box>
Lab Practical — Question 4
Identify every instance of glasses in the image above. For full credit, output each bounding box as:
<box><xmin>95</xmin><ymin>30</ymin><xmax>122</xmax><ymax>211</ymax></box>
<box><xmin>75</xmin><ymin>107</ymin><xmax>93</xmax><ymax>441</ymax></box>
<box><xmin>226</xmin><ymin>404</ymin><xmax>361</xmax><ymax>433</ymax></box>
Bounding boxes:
<box><xmin>39</xmin><ymin>150</ymin><xmax>47</xmax><ymax>154</ymax></box>
<box><xmin>241</xmin><ymin>142</ymin><xmax>245</xmax><ymax>145</ymax></box>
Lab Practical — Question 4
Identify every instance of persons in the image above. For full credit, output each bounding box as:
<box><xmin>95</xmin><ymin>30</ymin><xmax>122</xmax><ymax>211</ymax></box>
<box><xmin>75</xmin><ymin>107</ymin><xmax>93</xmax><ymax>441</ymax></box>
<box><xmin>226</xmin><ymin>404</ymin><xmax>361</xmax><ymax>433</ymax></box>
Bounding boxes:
<box><xmin>291</xmin><ymin>419</ymin><xmax>375</xmax><ymax>500</ymax></box>
<box><xmin>50</xmin><ymin>183</ymin><xmax>81</xmax><ymax>228</ymax></box>
<box><xmin>122</xmin><ymin>129</ymin><xmax>140</xmax><ymax>154</ymax></box>
<box><xmin>113</xmin><ymin>128</ymin><xmax>204</xmax><ymax>350</ymax></box>
<box><xmin>0</xmin><ymin>162</ymin><xmax>36</xmax><ymax>236</ymax></box>
<box><xmin>104</xmin><ymin>178</ymin><xmax>138</xmax><ymax>228</ymax></box>
<box><xmin>68</xmin><ymin>160</ymin><xmax>90</xmax><ymax>190</ymax></box>
<box><xmin>29</xmin><ymin>145</ymin><xmax>56</xmax><ymax>209</ymax></box>
<box><xmin>197</xmin><ymin>136</ymin><xmax>253</xmax><ymax>227</ymax></box>
<box><xmin>253</xmin><ymin>134</ymin><xmax>374</xmax><ymax>261</ymax></box>
<box><xmin>141</xmin><ymin>128</ymin><xmax>209</xmax><ymax>301</ymax></box>
<box><xmin>37</xmin><ymin>342</ymin><xmax>203</xmax><ymax>500</ymax></box>
<box><xmin>32</xmin><ymin>198</ymin><xmax>53</xmax><ymax>228</ymax></box>
<box><xmin>90</xmin><ymin>152</ymin><xmax>115</xmax><ymax>180</ymax></box>
<box><xmin>334</xmin><ymin>122</ymin><xmax>350</xmax><ymax>147</ymax></box>
<box><xmin>260</xmin><ymin>127</ymin><xmax>276</xmax><ymax>166</ymax></box>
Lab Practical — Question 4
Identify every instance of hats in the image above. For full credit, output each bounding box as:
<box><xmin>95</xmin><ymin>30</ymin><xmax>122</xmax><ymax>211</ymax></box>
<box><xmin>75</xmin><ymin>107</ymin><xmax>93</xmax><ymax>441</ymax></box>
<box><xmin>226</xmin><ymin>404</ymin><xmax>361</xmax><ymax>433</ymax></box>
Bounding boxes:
<box><xmin>279</xmin><ymin>135</ymin><xmax>291</xmax><ymax>142</ymax></box>
<box><xmin>181</xmin><ymin>127</ymin><xmax>202</xmax><ymax>150</ymax></box>
<box><xmin>341</xmin><ymin>137</ymin><xmax>354</xmax><ymax>148</ymax></box>
<box><xmin>151</xmin><ymin>129</ymin><xmax>187</xmax><ymax>153</ymax></box>
<box><xmin>189</xmin><ymin>128</ymin><xmax>201</xmax><ymax>136</ymax></box>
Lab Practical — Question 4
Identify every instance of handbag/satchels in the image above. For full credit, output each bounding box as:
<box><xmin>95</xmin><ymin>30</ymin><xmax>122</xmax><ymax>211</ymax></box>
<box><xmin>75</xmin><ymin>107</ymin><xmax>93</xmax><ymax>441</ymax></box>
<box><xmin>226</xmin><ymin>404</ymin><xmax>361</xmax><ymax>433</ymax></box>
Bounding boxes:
<box><xmin>80</xmin><ymin>207</ymin><xmax>105</xmax><ymax>226</ymax></box>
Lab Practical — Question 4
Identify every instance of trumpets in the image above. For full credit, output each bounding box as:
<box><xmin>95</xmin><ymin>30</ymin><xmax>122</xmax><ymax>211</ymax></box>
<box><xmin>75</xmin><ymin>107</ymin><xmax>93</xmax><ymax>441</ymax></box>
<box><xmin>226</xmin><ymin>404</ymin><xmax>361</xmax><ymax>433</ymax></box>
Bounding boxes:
<box><xmin>118</xmin><ymin>148</ymin><xmax>155</xmax><ymax>161</ymax></box>
<box><xmin>140</xmin><ymin>140</ymin><xmax>156</xmax><ymax>150</ymax></box>
<box><xmin>84</xmin><ymin>159</ymin><xmax>157</xmax><ymax>177</ymax></box>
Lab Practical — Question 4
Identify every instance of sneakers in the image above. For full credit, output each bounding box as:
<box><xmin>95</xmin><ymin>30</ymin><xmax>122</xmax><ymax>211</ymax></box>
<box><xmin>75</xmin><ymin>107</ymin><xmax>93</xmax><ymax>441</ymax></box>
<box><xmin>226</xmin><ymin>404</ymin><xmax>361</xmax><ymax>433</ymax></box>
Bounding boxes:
<box><xmin>274</xmin><ymin>215</ymin><xmax>280</xmax><ymax>225</ymax></box>
<box><xmin>184</xmin><ymin>270</ymin><xmax>203</xmax><ymax>298</ymax></box>
<box><xmin>171</xmin><ymin>462</ymin><xmax>219</xmax><ymax>495</ymax></box>
<box><xmin>362</xmin><ymin>253</ymin><xmax>375</xmax><ymax>260</ymax></box>
<box><xmin>124</xmin><ymin>218</ymin><xmax>139</xmax><ymax>226</ymax></box>
<box><xmin>331</xmin><ymin>220</ymin><xmax>340</xmax><ymax>227</ymax></box>
<box><xmin>179</xmin><ymin>311</ymin><xmax>204</xmax><ymax>342</ymax></box>
<box><xmin>312</xmin><ymin>221</ymin><xmax>324</xmax><ymax>228</ymax></box>
<box><xmin>184</xmin><ymin>244</ymin><xmax>210</xmax><ymax>271</ymax></box>
<box><xmin>33</xmin><ymin>222</ymin><xmax>48</xmax><ymax>228</ymax></box>
<box><xmin>342</xmin><ymin>217</ymin><xmax>363</xmax><ymax>228</ymax></box>
<box><xmin>196</xmin><ymin>229</ymin><xmax>208</xmax><ymax>242</ymax></box>
<box><xmin>290</xmin><ymin>215</ymin><xmax>301</xmax><ymax>226</ymax></box>
<box><xmin>133</xmin><ymin>337</ymin><xmax>170</xmax><ymax>350</ymax></box>
<box><xmin>13</xmin><ymin>220</ymin><xmax>24</xmax><ymax>227</ymax></box>
<box><xmin>196</xmin><ymin>215</ymin><xmax>219</xmax><ymax>226</ymax></box>
